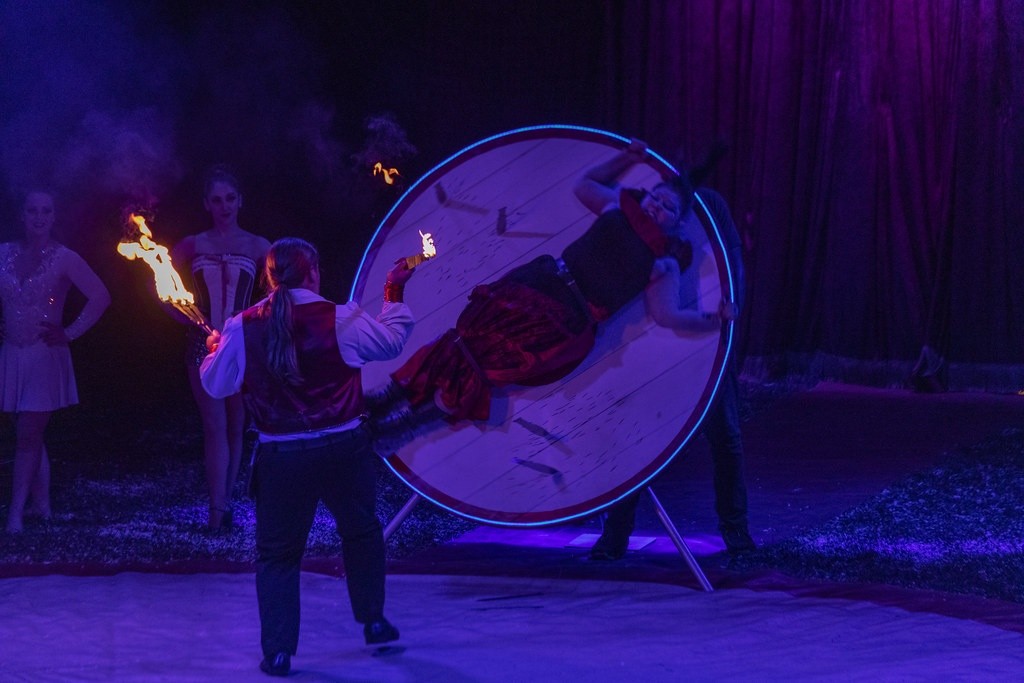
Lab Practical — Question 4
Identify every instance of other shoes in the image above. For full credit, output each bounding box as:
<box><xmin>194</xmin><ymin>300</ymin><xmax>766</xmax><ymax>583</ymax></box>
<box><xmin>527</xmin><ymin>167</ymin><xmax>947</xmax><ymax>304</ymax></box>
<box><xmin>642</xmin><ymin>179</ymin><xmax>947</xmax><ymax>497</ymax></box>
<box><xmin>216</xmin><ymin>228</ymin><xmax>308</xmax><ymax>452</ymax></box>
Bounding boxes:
<box><xmin>720</xmin><ymin>552</ymin><xmax>769</xmax><ymax>580</ymax></box>
<box><xmin>7</xmin><ymin>528</ymin><xmax>19</xmax><ymax>537</ymax></box>
<box><xmin>591</xmin><ymin>537</ymin><xmax>626</xmax><ymax>561</ymax></box>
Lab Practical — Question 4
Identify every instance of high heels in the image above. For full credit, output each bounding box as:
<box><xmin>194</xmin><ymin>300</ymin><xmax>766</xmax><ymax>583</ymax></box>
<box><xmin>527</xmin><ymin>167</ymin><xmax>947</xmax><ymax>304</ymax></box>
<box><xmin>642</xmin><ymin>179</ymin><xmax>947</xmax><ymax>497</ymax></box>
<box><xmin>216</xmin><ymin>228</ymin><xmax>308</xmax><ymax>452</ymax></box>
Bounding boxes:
<box><xmin>202</xmin><ymin>506</ymin><xmax>233</xmax><ymax>536</ymax></box>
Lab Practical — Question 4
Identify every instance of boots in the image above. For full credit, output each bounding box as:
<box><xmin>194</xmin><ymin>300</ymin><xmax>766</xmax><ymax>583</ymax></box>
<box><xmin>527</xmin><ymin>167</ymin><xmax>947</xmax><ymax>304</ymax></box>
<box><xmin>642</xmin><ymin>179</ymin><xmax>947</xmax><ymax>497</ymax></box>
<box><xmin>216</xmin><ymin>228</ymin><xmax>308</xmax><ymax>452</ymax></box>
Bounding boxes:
<box><xmin>360</xmin><ymin>399</ymin><xmax>416</xmax><ymax>441</ymax></box>
<box><xmin>364</xmin><ymin>380</ymin><xmax>404</xmax><ymax>417</ymax></box>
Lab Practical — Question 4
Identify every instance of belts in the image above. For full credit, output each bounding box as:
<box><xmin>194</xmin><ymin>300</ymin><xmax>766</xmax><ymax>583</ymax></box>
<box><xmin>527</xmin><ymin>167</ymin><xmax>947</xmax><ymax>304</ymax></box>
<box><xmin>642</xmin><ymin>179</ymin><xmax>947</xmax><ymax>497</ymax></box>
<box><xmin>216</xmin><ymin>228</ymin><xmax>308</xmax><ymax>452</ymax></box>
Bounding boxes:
<box><xmin>452</xmin><ymin>330</ymin><xmax>491</xmax><ymax>387</ymax></box>
<box><xmin>556</xmin><ymin>256</ymin><xmax>598</xmax><ymax>327</ymax></box>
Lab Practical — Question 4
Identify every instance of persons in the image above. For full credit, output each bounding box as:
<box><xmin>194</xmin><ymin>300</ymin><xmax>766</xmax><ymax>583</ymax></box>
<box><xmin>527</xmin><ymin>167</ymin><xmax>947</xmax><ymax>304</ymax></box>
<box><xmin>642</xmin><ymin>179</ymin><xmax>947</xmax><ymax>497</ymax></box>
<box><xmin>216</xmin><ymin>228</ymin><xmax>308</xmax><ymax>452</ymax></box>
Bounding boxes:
<box><xmin>0</xmin><ymin>187</ymin><xmax>111</xmax><ymax>536</ymax></box>
<box><xmin>198</xmin><ymin>237</ymin><xmax>401</xmax><ymax>674</ymax></box>
<box><xmin>153</xmin><ymin>169</ymin><xmax>272</xmax><ymax>535</ymax></box>
<box><xmin>591</xmin><ymin>159</ymin><xmax>758</xmax><ymax>556</ymax></box>
<box><xmin>362</xmin><ymin>136</ymin><xmax>736</xmax><ymax>440</ymax></box>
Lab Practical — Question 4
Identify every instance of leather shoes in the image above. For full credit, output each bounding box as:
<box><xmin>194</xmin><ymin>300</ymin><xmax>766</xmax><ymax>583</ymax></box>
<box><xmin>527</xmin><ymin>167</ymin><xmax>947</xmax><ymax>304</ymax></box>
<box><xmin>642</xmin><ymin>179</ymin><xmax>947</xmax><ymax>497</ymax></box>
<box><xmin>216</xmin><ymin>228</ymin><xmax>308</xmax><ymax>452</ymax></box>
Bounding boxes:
<box><xmin>258</xmin><ymin>651</ymin><xmax>291</xmax><ymax>677</ymax></box>
<box><xmin>363</xmin><ymin>617</ymin><xmax>400</xmax><ymax>645</ymax></box>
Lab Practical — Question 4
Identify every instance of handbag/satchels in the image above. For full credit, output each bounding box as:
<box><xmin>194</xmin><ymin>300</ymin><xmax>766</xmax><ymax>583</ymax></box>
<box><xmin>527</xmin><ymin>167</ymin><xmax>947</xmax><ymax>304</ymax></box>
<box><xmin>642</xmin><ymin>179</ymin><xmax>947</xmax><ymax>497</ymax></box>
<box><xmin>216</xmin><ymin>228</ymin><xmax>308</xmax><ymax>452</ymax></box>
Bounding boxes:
<box><xmin>246</xmin><ymin>438</ymin><xmax>260</xmax><ymax>497</ymax></box>
